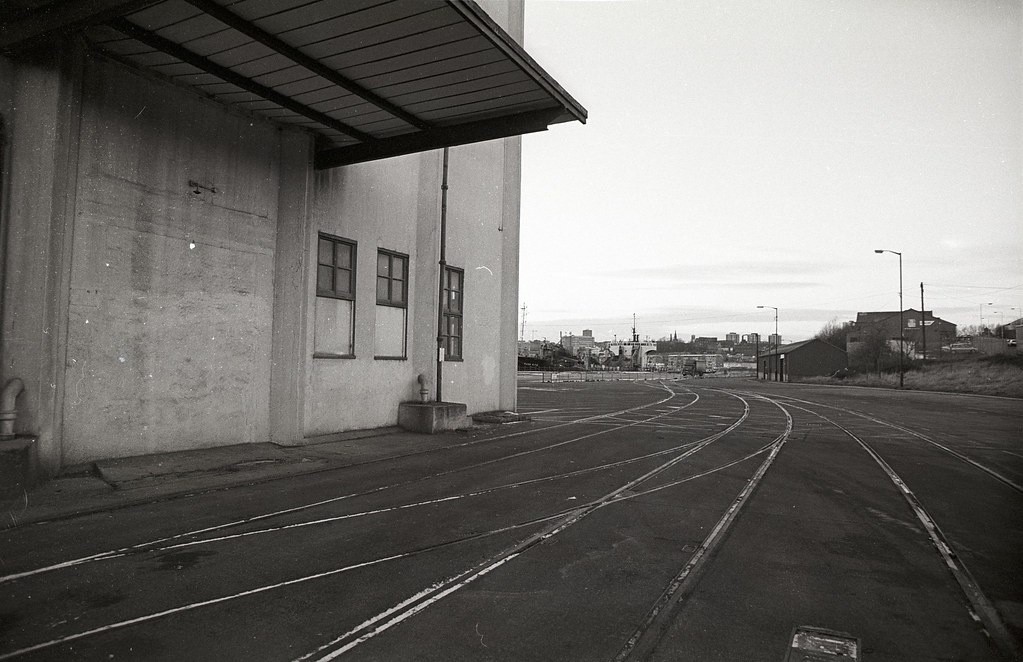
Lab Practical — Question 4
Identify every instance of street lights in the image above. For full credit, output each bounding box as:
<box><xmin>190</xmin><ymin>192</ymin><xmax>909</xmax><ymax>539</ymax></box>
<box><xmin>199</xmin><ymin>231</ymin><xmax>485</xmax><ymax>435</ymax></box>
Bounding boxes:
<box><xmin>757</xmin><ymin>306</ymin><xmax>778</xmax><ymax>382</ymax></box>
<box><xmin>874</xmin><ymin>249</ymin><xmax>904</xmax><ymax>388</ymax></box>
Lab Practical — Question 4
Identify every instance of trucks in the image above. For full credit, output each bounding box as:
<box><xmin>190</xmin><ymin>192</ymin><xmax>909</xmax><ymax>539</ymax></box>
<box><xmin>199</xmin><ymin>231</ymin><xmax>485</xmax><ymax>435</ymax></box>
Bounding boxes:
<box><xmin>682</xmin><ymin>360</ymin><xmax>706</xmax><ymax>376</ymax></box>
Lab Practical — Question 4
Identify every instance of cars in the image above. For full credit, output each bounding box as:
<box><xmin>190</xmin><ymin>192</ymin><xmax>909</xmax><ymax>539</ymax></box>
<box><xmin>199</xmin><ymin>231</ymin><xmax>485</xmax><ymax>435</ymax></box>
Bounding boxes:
<box><xmin>667</xmin><ymin>368</ymin><xmax>681</xmax><ymax>374</ymax></box>
